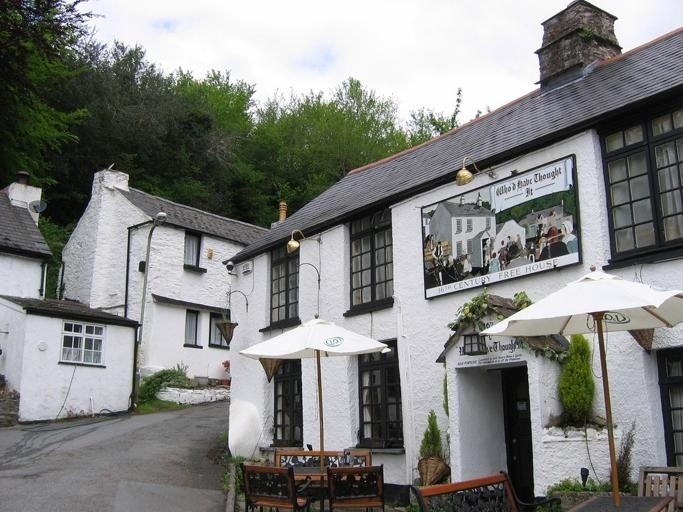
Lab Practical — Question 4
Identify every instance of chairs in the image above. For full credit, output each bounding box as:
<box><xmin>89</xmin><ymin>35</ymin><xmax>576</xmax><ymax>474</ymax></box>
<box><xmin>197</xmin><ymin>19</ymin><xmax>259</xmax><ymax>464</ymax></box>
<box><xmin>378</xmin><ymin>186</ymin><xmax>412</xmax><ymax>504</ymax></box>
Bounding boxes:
<box><xmin>239</xmin><ymin>463</ymin><xmax>312</xmax><ymax>512</ymax></box>
<box><xmin>637</xmin><ymin>466</ymin><xmax>683</xmax><ymax>512</ymax></box>
<box><xmin>327</xmin><ymin>465</ymin><xmax>384</xmax><ymax>512</ymax></box>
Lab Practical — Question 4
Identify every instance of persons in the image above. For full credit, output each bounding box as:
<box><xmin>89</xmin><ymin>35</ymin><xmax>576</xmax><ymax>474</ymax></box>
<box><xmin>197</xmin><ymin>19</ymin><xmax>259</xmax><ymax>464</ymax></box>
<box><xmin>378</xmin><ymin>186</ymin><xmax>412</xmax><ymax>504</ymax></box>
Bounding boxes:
<box><xmin>424</xmin><ymin>212</ymin><xmax>578</xmax><ymax>286</ymax></box>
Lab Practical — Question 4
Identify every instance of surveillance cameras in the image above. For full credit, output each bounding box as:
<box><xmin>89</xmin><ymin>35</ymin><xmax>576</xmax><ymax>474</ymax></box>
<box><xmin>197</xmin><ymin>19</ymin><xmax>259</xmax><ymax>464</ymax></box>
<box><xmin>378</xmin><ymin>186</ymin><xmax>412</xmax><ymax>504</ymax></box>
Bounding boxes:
<box><xmin>225</xmin><ymin>262</ymin><xmax>234</xmax><ymax>271</ymax></box>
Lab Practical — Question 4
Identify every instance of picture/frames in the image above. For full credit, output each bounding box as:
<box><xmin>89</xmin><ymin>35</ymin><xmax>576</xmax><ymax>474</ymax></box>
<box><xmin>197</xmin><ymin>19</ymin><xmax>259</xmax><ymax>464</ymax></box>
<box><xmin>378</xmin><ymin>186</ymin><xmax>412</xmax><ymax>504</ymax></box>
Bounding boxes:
<box><xmin>421</xmin><ymin>154</ymin><xmax>583</xmax><ymax>299</ymax></box>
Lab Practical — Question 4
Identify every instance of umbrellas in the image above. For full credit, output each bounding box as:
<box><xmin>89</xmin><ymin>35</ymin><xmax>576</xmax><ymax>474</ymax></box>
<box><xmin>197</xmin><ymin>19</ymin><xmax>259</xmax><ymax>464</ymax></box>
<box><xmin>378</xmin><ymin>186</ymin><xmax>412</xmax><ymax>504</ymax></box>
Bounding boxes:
<box><xmin>478</xmin><ymin>263</ymin><xmax>683</xmax><ymax>508</ymax></box>
<box><xmin>238</xmin><ymin>312</ymin><xmax>389</xmax><ymax>472</ymax></box>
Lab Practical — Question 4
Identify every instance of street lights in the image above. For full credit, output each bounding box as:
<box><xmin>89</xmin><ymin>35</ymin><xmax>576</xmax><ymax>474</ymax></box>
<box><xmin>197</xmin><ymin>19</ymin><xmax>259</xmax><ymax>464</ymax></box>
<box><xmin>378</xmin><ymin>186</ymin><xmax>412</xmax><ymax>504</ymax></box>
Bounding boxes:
<box><xmin>132</xmin><ymin>211</ymin><xmax>168</xmax><ymax>408</ymax></box>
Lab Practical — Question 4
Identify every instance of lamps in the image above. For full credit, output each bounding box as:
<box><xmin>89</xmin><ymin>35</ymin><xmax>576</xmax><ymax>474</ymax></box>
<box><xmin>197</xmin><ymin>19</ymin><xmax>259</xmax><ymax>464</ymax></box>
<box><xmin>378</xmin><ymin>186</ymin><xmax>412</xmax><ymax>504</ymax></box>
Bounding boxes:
<box><xmin>454</xmin><ymin>156</ymin><xmax>496</xmax><ymax>186</ymax></box>
<box><xmin>287</xmin><ymin>229</ymin><xmax>321</xmax><ymax>253</ymax></box>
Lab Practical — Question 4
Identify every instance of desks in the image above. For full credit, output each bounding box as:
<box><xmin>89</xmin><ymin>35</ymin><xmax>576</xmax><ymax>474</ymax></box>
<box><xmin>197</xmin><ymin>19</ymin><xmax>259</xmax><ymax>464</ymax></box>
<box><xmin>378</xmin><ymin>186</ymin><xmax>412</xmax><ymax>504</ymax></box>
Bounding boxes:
<box><xmin>294</xmin><ymin>472</ymin><xmax>329</xmax><ymax>512</ymax></box>
<box><xmin>566</xmin><ymin>495</ymin><xmax>673</xmax><ymax>512</ymax></box>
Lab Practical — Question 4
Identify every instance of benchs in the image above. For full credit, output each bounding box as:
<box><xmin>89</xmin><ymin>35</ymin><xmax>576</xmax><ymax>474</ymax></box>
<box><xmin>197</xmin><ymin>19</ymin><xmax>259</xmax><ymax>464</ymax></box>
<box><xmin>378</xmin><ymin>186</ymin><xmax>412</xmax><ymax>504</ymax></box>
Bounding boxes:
<box><xmin>273</xmin><ymin>448</ymin><xmax>373</xmax><ymax>512</ymax></box>
<box><xmin>410</xmin><ymin>471</ymin><xmax>562</xmax><ymax>512</ymax></box>
